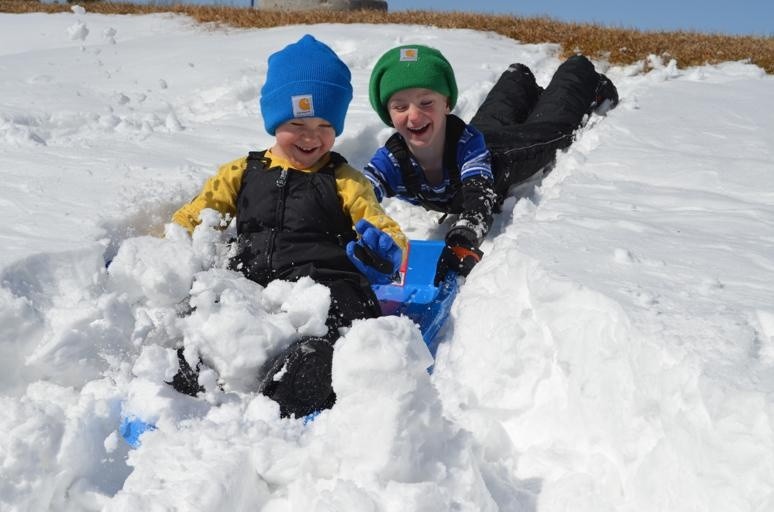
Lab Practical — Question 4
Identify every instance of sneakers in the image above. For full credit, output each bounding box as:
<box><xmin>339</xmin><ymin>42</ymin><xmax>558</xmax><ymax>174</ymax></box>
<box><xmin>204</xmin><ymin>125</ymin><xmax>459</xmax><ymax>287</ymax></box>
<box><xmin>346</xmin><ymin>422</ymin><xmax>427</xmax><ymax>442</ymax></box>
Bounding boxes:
<box><xmin>492</xmin><ymin>62</ymin><xmax>545</xmax><ymax>99</ymax></box>
<box><xmin>555</xmin><ymin>53</ymin><xmax>619</xmax><ymax>115</ymax></box>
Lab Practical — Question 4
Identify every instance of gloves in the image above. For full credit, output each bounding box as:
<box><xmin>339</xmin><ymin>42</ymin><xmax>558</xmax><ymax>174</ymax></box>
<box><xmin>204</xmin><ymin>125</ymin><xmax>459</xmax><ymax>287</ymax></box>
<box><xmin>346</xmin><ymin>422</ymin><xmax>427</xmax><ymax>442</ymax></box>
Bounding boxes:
<box><xmin>343</xmin><ymin>218</ymin><xmax>401</xmax><ymax>287</ymax></box>
<box><xmin>432</xmin><ymin>225</ymin><xmax>484</xmax><ymax>289</ymax></box>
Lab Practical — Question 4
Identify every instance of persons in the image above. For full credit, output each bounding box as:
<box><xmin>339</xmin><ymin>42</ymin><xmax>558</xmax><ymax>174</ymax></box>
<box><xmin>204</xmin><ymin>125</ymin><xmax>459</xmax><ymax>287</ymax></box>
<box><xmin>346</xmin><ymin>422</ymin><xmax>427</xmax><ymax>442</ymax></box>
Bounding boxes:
<box><xmin>361</xmin><ymin>43</ymin><xmax>619</xmax><ymax>273</ymax></box>
<box><xmin>159</xmin><ymin>32</ymin><xmax>412</xmax><ymax>420</ymax></box>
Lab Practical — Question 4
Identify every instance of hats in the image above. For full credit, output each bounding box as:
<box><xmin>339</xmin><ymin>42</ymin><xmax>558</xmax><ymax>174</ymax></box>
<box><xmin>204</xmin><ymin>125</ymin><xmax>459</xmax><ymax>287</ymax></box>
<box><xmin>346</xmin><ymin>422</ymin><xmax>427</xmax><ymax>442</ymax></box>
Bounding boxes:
<box><xmin>366</xmin><ymin>44</ymin><xmax>459</xmax><ymax>129</ymax></box>
<box><xmin>258</xmin><ymin>33</ymin><xmax>355</xmax><ymax>138</ymax></box>
